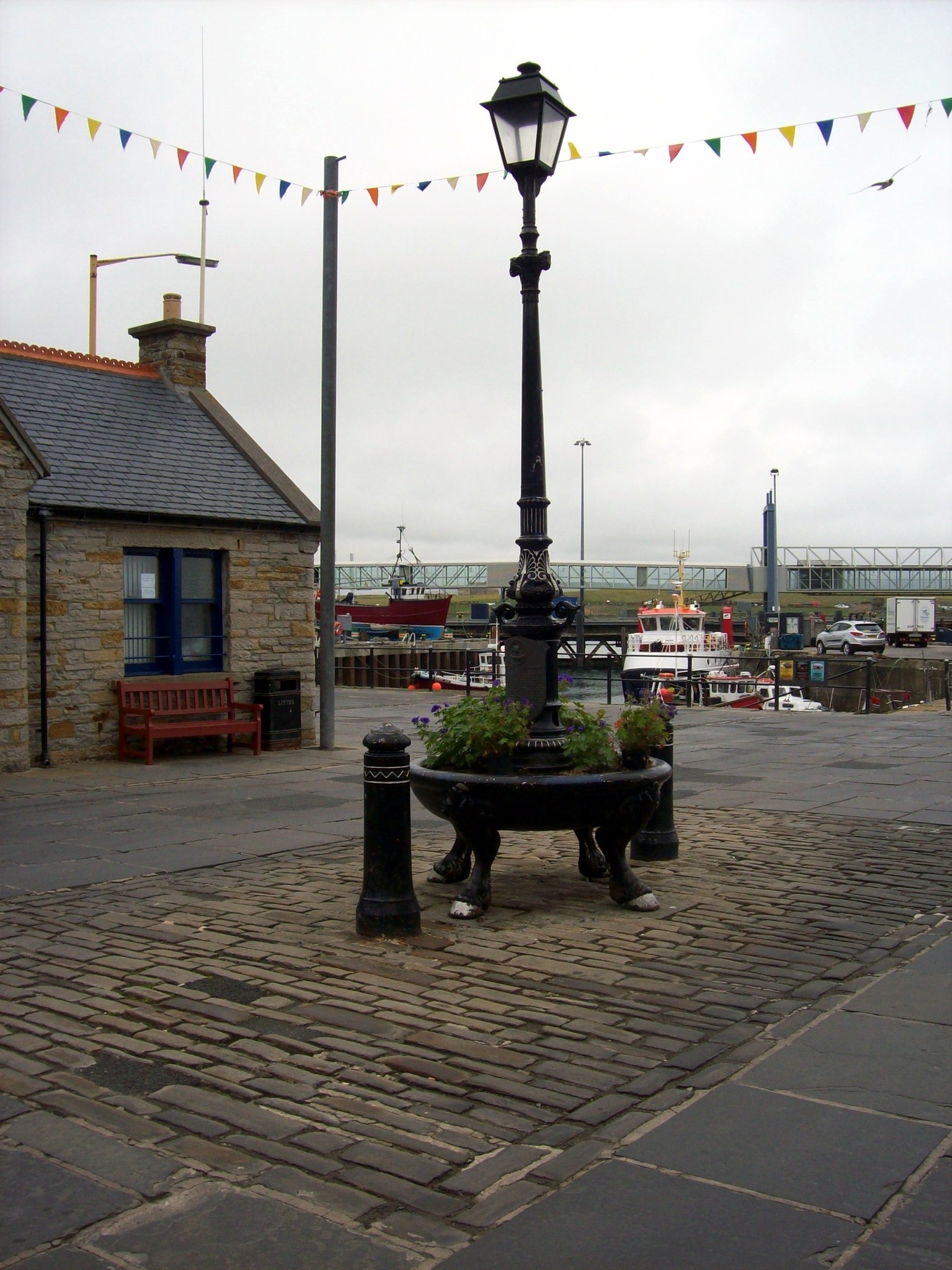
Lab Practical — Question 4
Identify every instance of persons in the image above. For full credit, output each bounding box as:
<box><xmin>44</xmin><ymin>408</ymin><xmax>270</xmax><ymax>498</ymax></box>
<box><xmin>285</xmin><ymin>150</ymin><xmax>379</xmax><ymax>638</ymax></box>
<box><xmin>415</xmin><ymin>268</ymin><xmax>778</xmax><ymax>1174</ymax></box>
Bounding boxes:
<box><xmin>340</xmin><ymin>593</ymin><xmax>353</xmax><ymax>604</ymax></box>
<box><xmin>388</xmin><ymin>574</ymin><xmax>405</xmax><ymax>600</ymax></box>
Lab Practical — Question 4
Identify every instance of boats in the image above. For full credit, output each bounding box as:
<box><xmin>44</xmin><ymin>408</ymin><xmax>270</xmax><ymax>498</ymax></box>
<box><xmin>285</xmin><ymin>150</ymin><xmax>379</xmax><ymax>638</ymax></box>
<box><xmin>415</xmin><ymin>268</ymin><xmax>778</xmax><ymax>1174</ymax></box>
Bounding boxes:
<box><xmin>619</xmin><ymin>529</ymin><xmax>740</xmax><ymax>704</ymax></box>
<box><xmin>411</xmin><ymin>645</ymin><xmax>505</xmax><ymax>690</ymax></box>
<box><xmin>640</xmin><ymin>672</ymin><xmax>831</xmax><ymax>713</ymax></box>
<box><xmin>315</xmin><ymin>578</ymin><xmax>454</xmax><ymax>641</ymax></box>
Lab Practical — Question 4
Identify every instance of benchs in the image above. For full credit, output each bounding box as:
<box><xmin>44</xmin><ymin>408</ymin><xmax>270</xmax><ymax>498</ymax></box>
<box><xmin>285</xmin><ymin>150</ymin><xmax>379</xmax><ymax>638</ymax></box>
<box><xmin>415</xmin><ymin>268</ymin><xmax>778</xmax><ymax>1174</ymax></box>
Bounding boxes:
<box><xmin>117</xmin><ymin>677</ymin><xmax>263</xmax><ymax>765</ymax></box>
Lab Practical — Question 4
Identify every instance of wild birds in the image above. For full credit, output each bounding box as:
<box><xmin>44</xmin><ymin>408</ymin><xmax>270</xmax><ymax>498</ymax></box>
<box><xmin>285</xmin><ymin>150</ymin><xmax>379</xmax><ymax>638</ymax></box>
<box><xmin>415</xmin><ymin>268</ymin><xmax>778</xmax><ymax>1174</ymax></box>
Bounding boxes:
<box><xmin>850</xmin><ymin>154</ymin><xmax>921</xmax><ymax>195</ymax></box>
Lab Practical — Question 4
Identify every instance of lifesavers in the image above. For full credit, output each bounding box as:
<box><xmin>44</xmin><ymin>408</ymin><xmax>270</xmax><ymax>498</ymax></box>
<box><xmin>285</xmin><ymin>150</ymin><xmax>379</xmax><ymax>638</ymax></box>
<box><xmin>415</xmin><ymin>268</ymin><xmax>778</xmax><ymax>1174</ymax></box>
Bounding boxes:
<box><xmin>756</xmin><ymin>678</ymin><xmax>773</xmax><ymax>683</ymax></box>
<box><xmin>334</xmin><ymin>622</ymin><xmax>342</xmax><ymax>635</ymax></box>
<box><xmin>744</xmin><ymin>622</ymin><xmax>748</xmax><ymax>635</ymax></box>
<box><xmin>658</xmin><ymin>672</ymin><xmax>675</xmax><ymax>678</ymax></box>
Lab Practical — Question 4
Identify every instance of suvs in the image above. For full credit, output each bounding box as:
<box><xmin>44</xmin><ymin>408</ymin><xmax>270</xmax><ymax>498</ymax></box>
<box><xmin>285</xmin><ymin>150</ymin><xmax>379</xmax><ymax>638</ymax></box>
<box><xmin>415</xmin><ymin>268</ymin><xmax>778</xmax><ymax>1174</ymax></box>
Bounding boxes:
<box><xmin>816</xmin><ymin>620</ymin><xmax>886</xmax><ymax>654</ymax></box>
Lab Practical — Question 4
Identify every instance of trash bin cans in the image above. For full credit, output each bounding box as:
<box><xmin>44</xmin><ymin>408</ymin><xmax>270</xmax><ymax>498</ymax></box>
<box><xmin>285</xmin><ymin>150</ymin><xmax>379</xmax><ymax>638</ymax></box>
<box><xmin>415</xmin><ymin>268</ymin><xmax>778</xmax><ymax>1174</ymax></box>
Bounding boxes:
<box><xmin>253</xmin><ymin>667</ymin><xmax>304</xmax><ymax>753</ymax></box>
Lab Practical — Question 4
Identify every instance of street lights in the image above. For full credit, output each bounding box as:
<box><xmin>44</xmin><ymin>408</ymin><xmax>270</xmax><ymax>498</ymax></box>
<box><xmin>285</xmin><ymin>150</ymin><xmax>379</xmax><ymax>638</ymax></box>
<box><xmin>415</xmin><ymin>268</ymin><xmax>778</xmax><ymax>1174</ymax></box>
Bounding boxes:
<box><xmin>389</xmin><ymin>526</ymin><xmax>407</xmax><ymax>584</ymax></box>
<box><xmin>480</xmin><ymin>60</ymin><xmax>579</xmax><ymax>722</ymax></box>
<box><xmin>771</xmin><ymin>467</ymin><xmax>780</xmax><ymax>639</ymax></box>
<box><xmin>88</xmin><ymin>252</ymin><xmax>220</xmax><ymax>357</ymax></box>
<box><xmin>574</xmin><ymin>440</ymin><xmax>592</xmax><ymax>667</ymax></box>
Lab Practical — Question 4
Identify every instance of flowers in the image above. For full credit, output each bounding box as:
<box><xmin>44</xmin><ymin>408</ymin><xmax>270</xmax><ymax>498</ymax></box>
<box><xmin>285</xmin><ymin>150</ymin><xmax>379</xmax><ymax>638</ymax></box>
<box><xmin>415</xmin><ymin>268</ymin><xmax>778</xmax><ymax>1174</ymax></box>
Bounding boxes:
<box><xmin>411</xmin><ymin>673</ymin><xmax>679</xmax><ymax>776</ymax></box>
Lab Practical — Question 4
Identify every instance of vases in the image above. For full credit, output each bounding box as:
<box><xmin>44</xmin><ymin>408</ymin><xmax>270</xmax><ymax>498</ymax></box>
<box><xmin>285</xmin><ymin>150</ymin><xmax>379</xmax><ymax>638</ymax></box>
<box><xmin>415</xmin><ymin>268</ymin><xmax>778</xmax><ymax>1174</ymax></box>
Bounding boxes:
<box><xmin>411</xmin><ymin>754</ymin><xmax>671</xmax><ymax>918</ymax></box>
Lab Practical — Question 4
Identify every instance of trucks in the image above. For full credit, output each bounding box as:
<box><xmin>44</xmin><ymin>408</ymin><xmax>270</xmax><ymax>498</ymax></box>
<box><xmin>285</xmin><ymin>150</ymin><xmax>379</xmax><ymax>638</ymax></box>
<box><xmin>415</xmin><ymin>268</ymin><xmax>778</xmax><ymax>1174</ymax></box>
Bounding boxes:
<box><xmin>885</xmin><ymin>597</ymin><xmax>936</xmax><ymax>648</ymax></box>
